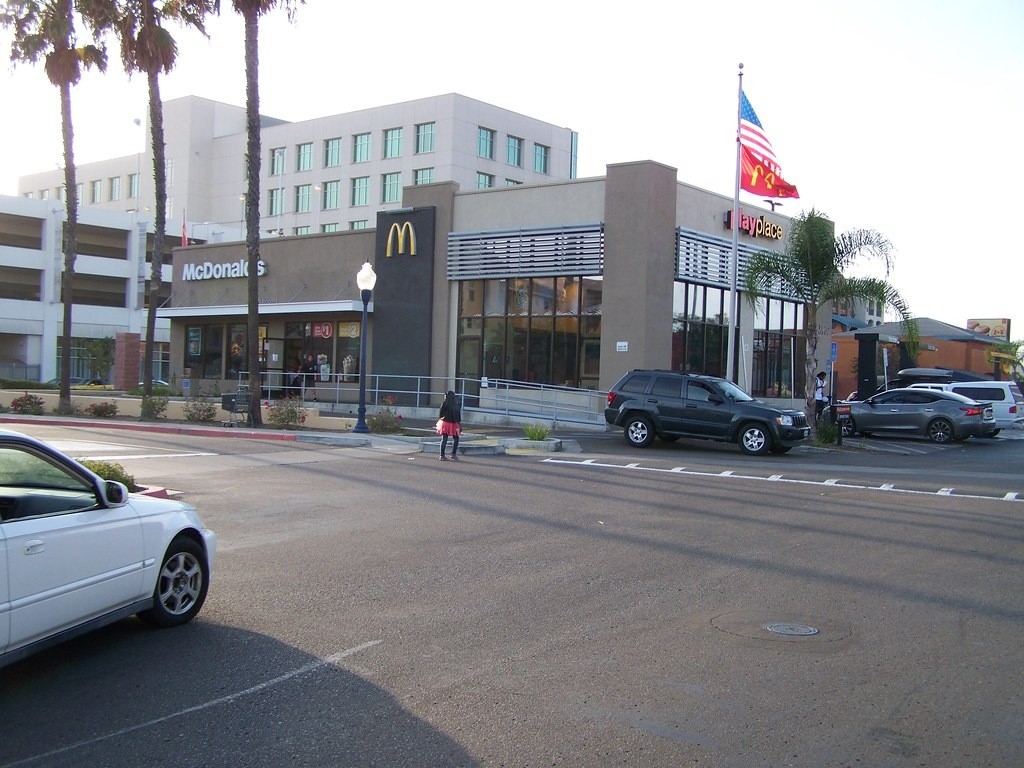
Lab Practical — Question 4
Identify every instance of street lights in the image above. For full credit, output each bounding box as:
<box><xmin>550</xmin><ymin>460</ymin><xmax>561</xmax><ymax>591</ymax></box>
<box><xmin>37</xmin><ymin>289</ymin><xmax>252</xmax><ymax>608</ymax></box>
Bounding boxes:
<box><xmin>352</xmin><ymin>258</ymin><xmax>376</xmax><ymax>433</ymax></box>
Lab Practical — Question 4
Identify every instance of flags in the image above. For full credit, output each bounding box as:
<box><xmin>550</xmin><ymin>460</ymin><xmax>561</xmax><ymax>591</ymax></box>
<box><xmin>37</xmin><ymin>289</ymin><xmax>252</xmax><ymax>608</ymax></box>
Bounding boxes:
<box><xmin>739</xmin><ymin>142</ymin><xmax>801</xmax><ymax>198</ymax></box>
<box><xmin>740</xmin><ymin>88</ymin><xmax>784</xmax><ymax>172</ymax></box>
<box><xmin>181</xmin><ymin>208</ymin><xmax>188</xmax><ymax>246</ymax></box>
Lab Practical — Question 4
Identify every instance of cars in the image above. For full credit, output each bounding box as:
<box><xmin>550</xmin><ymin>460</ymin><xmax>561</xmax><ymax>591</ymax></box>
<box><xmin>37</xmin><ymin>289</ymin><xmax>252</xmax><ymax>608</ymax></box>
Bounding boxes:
<box><xmin>48</xmin><ymin>376</ymin><xmax>107</xmax><ymax>389</ymax></box>
<box><xmin>138</xmin><ymin>379</ymin><xmax>169</xmax><ymax>386</ymax></box>
<box><xmin>816</xmin><ymin>388</ymin><xmax>995</xmax><ymax>445</ymax></box>
<box><xmin>0</xmin><ymin>429</ymin><xmax>215</xmax><ymax>668</ymax></box>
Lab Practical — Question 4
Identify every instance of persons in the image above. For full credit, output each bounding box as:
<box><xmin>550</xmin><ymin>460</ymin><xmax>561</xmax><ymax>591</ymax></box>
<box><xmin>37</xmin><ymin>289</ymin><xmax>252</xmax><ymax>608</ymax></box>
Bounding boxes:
<box><xmin>814</xmin><ymin>372</ymin><xmax>827</xmax><ymax>421</ymax></box>
<box><xmin>296</xmin><ymin>354</ymin><xmax>319</xmax><ymax>402</ymax></box>
<box><xmin>435</xmin><ymin>390</ymin><xmax>463</xmax><ymax>461</ymax></box>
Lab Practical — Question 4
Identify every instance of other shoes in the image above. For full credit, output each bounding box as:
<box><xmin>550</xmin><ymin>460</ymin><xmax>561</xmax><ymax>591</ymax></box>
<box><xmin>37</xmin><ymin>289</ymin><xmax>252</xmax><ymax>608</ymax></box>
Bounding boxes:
<box><xmin>313</xmin><ymin>398</ymin><xmax>319</xmax><ymax>402</ymax></box>
<box><xmin>439</xmin><ymin>456</ymin><xmax>448</xmax><ymax>461</ymax></box>
<box><xmin>450</xmin><ymin>454</ymin><xmax>458</xmax><ymax>460</ymax></box>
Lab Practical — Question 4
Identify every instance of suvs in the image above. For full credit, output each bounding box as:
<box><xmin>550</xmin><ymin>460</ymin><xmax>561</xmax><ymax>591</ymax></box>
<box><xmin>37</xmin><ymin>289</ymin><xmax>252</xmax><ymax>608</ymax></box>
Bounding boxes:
<box><xmin>847</xmin><ymin>367</ymin><xmax>993</xmax><ymax>401</ymax></box>
<box><xmin>847</xmin><ymin>377</ymin><xmax>1024</xmax><ymax>438</ymax></box>
<box><xmin>604</xmin><ymin>369</ymin><xmax>811</xmax><ymax>456</ymax></box>
